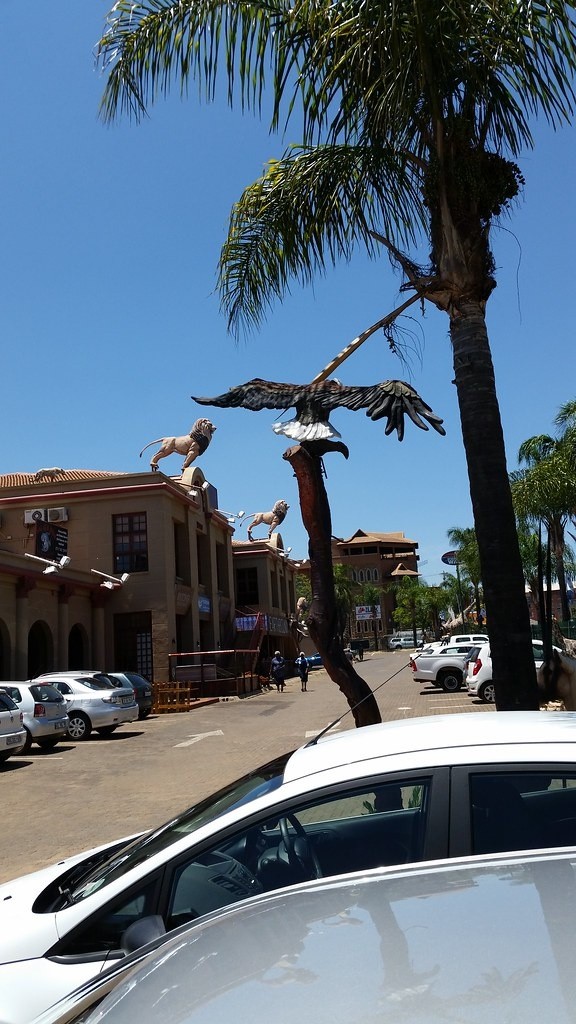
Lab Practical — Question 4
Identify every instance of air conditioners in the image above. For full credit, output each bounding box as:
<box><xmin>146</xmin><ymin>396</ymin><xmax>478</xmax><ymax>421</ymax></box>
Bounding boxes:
<box><xmin>48</xmin><ymin>507</ymin><xmax>68</xmax><ymax>523</ymax></box>
<box><xmin>25</xmin><ymin>509</ymin><xmax>47</xmax><ymax>524</ymax></box>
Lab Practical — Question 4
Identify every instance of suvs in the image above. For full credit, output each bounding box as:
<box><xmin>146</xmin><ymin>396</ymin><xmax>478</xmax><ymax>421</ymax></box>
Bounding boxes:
<box><xmin>387</xmin><ymin>637</ymin><xmax>424</xmax><ymax>651</ymax></box>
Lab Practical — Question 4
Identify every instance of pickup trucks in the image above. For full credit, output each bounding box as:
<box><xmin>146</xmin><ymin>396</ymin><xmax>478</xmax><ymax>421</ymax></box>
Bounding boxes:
<box><xmin>417</xmin><ymin>634</ymin><xmax>488</xmax><ymax>651</ymax></box>
<box><xmin>409</xmin><ymin>640</ymin><xmax>489</xmax><ymax>694</ymax></box>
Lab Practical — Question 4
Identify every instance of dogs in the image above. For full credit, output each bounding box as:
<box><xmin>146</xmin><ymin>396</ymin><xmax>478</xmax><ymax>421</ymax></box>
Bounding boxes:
<box><xmin>29</xmin><ymin>468</ymin><xmax>66</xmax><ymax>482</ymax></box>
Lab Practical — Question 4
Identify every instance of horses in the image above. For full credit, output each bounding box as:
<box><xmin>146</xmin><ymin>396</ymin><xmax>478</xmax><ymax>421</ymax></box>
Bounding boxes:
<box><xmin>538</xmin><ymin>648</ymin><xmax>576</xmax><ymax>712</ymax></box>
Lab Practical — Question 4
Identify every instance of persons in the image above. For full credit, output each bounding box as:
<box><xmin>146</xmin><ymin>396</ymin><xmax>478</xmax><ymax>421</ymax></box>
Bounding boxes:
<box><xmin>359</xmin><ymin>644</ymin><xmax>363</xmax><ymax>660</ymax></box>
<box><xmin>269</xmin><ymin>651</ymin><xmax>286</xmax><ymax>693</ymax></box>
<box><xmin>294</xmin><ymin>652</ymin><xmax>308</xmax><ymax>692</ymax></box>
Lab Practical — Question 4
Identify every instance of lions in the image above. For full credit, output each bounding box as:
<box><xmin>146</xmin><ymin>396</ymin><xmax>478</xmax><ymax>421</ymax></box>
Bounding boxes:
<box><xmin>296</xmin><ymin>596</ymin><xmax>309</xmax><ymax>621</ymax></box>
<box><xmin>240</xmin><ymin>500</ymin><xmax>291</xmax><ymax>542</ymax></box>
<box><xmin>139</xmin><ymin>418</ymin><xmax>217</xmax><ymax>476</ymax></box>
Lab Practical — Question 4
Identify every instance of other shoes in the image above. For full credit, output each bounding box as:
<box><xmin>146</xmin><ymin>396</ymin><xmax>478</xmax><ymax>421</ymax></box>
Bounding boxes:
<box><xmin>281</xmin><ymin>689</ymin><xmax>284</xmax><ymax>692</ymax></box>
<box><xmin>303</xmin><ymin>689</ymin><xmax>307</xmax><ymax>692</ymax></box>
<box><xmin>278</xmin><ymin>690</ymin><xmax>280</xmax><ymax>693</ymax></box>
<box><xmin>301</xmin><ymin>689</ymin><xmax>304</xmax><ymax>692</ymax></box>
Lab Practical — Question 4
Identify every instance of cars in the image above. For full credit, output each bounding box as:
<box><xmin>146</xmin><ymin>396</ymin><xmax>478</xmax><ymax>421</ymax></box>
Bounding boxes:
<box><xmin>0</xmin><ymin>681</ymin><xmax>70</xmax><ymax>756</ymax></box>
<box><xmin>36</xmin><ymin>670</ymin><xmax>138</xmax><ymax>705</ymax></box>
<box><xmin>24</xmin><ymin>676</ymin><xmax>139</xmax><ymax>740</ymax></box>
<box><xmin>294</xmin><ymin>648</ymin><xmax>355</xmax><ymax>667</ymax></box>
<box><xmin>0</xmin><ymin>688</ymin><xmax>27</xmax><ymax>764</ymax></box>
<box><xmin>0</xmin><ymin>711</ymin><xmax>576</xmax><ymax>1023</ymax></box>
<box><xmin>461</xmin><ymin>638</ymin><xmax>567</xmax><ymax>703</ymax></box>
<box><xmin>101</xmin><ymin>672</ymin><xmax>158</xmax><ymax>720</ymax></box>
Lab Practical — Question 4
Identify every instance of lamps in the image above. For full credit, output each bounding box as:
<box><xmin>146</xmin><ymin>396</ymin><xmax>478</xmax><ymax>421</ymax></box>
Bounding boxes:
<box><xmin>288</xmin><ymin>558</ymin><xmax>306</xmax><ymax>567</ymax></box>
<box><xmin>23</xmin><ymin>552</ymin><xmax>71</xmax><ymax>574</ymax></box>
<box><xmin>272</xmin><ymin>547</ymin><xmax>292</xmax><ymax>557</ymax></box>
<box><xmin>215</xmin><ymin>509</ymin><xmax>245</xmax><ymax>523</ymax></box>
<box><xmin>90</xmin><ymin>568</ymin><xmax>130</xmax><ymax>590</ymax></box>
<box><xmin>175</xmin><ymin>481</ymin><xmax>209</xmax><ymax>497</ymax></box>
